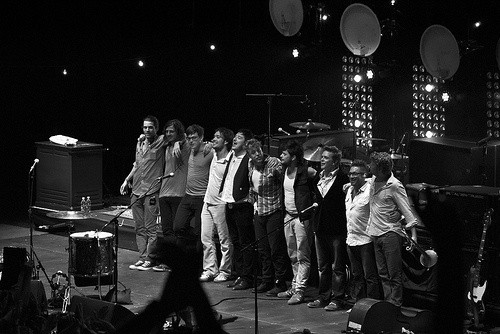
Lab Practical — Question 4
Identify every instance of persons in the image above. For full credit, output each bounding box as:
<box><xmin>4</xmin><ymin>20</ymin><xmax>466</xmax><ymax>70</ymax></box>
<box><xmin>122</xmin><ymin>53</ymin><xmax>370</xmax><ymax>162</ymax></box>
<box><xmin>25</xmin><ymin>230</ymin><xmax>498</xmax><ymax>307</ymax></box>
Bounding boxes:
<box><xmin>344</xmin><ymin>162</ymin><xmax>381</xmax><ymax>314</ymax></box>
<box><xmin>246</xmin><ymin>139</ymin><xmax>317</xmax><ymax>297</ymax></box>
<box><xmin>220</xmin><ymin>131</ymin><xmax>251</xmax><ymax>290</ymax></box>
<box><xmin>182</xmin><ymin>125</ymin><xmax>233</xmax><ymax>283</ymax></box>
<box><xmin>307</xmin><ymin>145</ymin><xmax>350</xmax><ymax>310</ymax></box>
<box><xmin>125</xmin><ymin>117</ymin><xmax>190</xmax><ymax>273</ymax></box>
<box><xmin>343</xmin><ymin>150</ymin><xmax>424</xmax><ymax>321</ymax></box>
<box><xmin>277</xmin><ymin>140</ymin><xmax>312</xmax><ymax>305</ymax></box>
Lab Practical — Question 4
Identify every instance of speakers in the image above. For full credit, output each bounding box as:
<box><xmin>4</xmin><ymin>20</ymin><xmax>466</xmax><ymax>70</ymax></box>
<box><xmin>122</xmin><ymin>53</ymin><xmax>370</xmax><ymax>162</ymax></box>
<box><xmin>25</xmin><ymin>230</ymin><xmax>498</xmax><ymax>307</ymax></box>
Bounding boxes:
<box><xmin>69</xmin><ymin>295</ymin><xmax>136</xmax><ymax>330</ymax></box>
<box><xmin>437</xmin><ymin>186</ymin><xmax>500</xmax><ymax>249</ymax></box>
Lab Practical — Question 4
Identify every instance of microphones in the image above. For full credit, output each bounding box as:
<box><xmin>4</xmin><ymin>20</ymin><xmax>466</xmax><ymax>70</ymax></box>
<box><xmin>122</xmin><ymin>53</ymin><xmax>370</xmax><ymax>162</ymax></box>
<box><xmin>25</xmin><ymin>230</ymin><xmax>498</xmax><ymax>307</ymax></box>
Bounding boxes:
<box><xmin>29</xmin><ymin>158</ymin><xmax>39</xmax><ymax>172</ymax></box>
<box><xmin>158</xmin><ymin>172</ymin><xmax>174</xmax><ymax>179</ymax></box>
<box><xmin>300</xmin><ymin>202</ymin><xmax>318</xmax><ymax>213</ymax></box>
<box><xmin>396</xmin><ymin>133</ymin><xmax>405</xmax><ymax>153</ymax></box>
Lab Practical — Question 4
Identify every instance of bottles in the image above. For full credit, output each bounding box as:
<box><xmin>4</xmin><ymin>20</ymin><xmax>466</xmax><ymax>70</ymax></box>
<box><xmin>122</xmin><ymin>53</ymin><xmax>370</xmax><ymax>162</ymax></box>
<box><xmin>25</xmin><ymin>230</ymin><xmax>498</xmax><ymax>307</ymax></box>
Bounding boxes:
<box><xmin>85</xmin><ymin>196</ymin><xmax>91</xmax><ymax>213</ymax></box>
<box><xmin>80</xmin><ymin>197</ymin><xmax>86</xmax><ymax>213</ymax></box>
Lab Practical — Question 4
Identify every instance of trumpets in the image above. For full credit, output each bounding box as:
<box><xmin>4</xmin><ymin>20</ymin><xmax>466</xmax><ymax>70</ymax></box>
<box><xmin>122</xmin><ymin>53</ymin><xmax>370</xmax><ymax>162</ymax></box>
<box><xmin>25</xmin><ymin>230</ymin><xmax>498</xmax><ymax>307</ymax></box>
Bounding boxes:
<box><xmin>400</xmin><ymin>229</ymin><xmax>438</xmax><ymax>268</ymax></box>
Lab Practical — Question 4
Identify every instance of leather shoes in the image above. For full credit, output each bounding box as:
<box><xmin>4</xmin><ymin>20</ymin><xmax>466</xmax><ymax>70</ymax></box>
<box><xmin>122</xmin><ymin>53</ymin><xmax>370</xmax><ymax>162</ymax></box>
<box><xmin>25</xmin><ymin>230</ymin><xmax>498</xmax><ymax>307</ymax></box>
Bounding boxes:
<box><xmin>233</xmin><ymin>280</ymin><xmax>250</xmax><ymax>290</ymax></box>
<box><xmin>226</xmin><ymin>277</ymin><xmax>243</xmax><ymax>287</ymax></box>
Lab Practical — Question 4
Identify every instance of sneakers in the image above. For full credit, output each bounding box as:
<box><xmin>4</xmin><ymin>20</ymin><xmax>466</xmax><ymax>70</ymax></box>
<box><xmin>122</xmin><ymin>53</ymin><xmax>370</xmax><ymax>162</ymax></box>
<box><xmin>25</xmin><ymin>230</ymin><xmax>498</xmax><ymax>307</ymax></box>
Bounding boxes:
<box><xmin>137</xmin><ymin>261</ymin><xmax>153</xmax><ymax>271</ymax></box>
<box><xmin>129</xmin><ymin>259</ymin><xmax>143</xmax><ymax>270</ymax></box>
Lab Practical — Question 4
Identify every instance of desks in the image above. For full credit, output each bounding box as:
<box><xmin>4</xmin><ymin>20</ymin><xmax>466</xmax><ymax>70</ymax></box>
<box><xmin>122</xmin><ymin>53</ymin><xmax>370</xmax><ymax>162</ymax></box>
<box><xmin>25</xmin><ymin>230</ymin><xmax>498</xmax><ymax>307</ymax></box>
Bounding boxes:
<box><xmin>35</xmin><ymin>142</ymin><xmax>104</xmax><ymax>209</ymax></box>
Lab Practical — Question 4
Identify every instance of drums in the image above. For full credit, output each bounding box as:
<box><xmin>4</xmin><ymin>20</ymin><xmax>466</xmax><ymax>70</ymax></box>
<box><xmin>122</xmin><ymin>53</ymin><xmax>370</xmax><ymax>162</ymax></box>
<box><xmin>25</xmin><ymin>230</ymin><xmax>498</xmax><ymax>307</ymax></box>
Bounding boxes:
<box><xmin>67</xmin><ymin>231</ymin><xmax>115</xmax><ymax>278</ymax></box>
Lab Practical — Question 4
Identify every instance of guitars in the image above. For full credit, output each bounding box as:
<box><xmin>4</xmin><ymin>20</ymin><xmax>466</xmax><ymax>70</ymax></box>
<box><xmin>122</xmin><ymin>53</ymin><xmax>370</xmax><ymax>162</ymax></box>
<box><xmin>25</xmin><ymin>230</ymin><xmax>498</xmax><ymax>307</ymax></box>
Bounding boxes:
<box><xmin>458</xmin><ymin>208</ymin><xmax>494</xmax><ymax>323</ymax></box>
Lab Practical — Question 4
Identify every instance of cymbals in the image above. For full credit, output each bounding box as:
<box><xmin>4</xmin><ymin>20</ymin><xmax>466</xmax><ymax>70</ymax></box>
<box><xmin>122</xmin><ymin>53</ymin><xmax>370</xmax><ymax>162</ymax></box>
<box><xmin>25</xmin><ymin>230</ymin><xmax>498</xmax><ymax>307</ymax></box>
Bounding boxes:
<box><xmin>350</xmin><ymin>137</ymin><xmax>387</xmax><ymax>141</ymax></box>
<box><xmin>289</xmin><ymin>122</ymin><xmax>330</xmax><ymax>130</ymax></box>
<box><xmin>390</xmin><ymin>154</ymin><xmax>409</xmax><ymax>160</ymax></box>
<box><xmin>46</xmin><ymin>210</ymin><xmax>103</xmax><ymax>220</ymax></box>
<box><xmin>302</xmin><ymin>137</ymin><xmax>344</xmax><ymax>161</ymax></box>
<box><xmin>339</xmin><ymin>159</ymin><xmax>354</xmax><ymax>166</ymax></box>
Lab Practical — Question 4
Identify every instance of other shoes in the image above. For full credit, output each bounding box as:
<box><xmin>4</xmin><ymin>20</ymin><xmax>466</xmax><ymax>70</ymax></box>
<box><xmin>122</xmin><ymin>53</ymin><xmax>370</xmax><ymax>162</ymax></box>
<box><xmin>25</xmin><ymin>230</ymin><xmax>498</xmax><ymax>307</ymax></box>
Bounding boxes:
<box><xmin>277</xmin><ymin>289</ymin><xmax>295</xmax><ymax>298</ymax></box>
<box><xmin>287</xmin><ymin>291</ymin><xmax>304</xmax><ymax>305</ymax></box>
<box><xmin>265</xmin><ymin>286</ymin><xmax>287</xmax><ymax>296</ymax></box>
<box><xmin>199</xmin><ymin>274</ymin><xmax>211</xmax><ymax>281</ymax></box>
<box><xmin>251</xmin><ymin>283</ymin><xmax>274</xmax><ymax>293</ymax></box>
<box><xmin>324</xmin><ymin>301</ymin><xmax>342</xmax><ymax>311</ymax></box>
<box><xmin>153</xmin><ymin>264</ymin><xmax>167</xmax><ymax>271</ymax></box>
<box><xmin>307</xmin><ymin>299</ymin><xmax>324</xmax><ymax>308</ymax></box>
<box><xmin>213</xmin><ymin>271</ymin><xmax>231</xmax><ymax>283</ymax></box>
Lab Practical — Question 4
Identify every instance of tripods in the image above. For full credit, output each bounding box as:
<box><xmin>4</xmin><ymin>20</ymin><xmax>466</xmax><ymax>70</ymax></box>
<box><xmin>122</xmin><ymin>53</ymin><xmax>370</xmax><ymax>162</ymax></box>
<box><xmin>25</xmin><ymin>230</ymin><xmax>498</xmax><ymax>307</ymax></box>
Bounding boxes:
<box><xmin>27</xmin><ymin>170</ymin><xmax>52</xmax><ymax>287</ymax></box>
